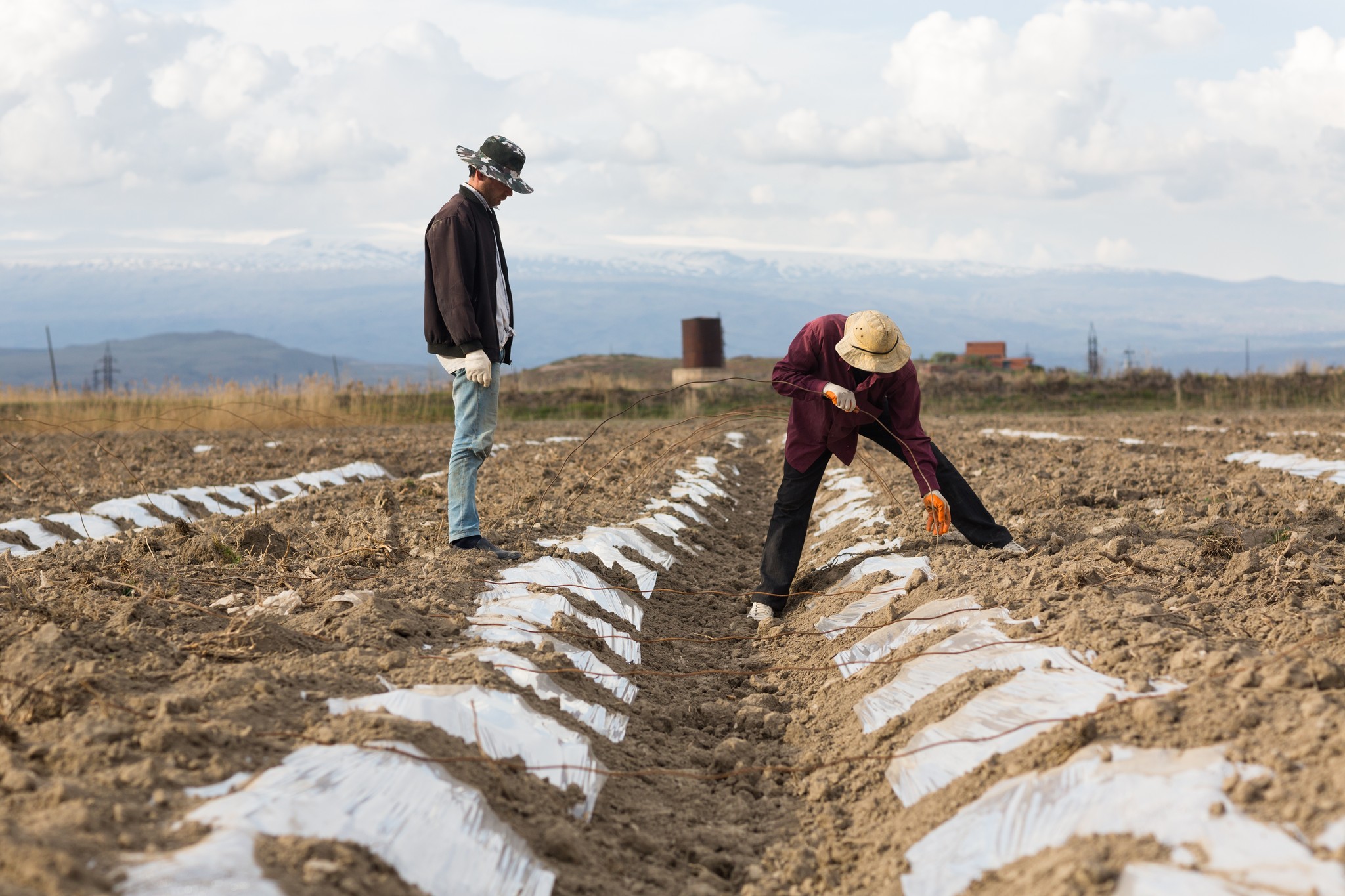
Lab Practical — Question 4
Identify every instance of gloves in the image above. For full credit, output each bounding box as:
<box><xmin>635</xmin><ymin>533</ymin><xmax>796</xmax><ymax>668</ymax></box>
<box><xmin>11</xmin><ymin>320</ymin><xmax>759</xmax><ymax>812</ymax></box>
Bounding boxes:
<box><xmin>923</xmin><ymin>490</ymin><xmax>950</xmax><ymax>536</ymax></box>
<box><xmin>465</xmin><ymin>349</ymin><xmax>492</xmax><ymax>388</ymax></box>
<box><xmin>823</xmin><ymin>381</ymin><xmax>857</xmax><ymax>413</ymax></box>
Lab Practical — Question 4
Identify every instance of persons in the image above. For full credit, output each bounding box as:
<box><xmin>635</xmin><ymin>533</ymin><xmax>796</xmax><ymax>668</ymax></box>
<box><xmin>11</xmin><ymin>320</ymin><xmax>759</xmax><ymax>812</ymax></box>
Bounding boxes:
<box><xmin>423</xmin><ymin>136</ymin><xmax>535</xmax><ymax>562</ymax></box>
<box><xmin>746</xmin><ymin>309</ymin><xmax>1030</xmax><ymax>629</ymax></box>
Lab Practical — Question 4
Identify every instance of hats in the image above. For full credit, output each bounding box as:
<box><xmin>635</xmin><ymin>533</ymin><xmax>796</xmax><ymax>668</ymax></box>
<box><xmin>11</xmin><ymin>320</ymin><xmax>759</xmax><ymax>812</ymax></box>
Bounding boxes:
<box><xmin>457</xmin><ymin>135</ymin><xmax>534</xmax><ymax>193</ymax></box>
<box><xmin>835</xmin><ymin>310</ymin><xmax>911</xmax><ymax>373</ymax></box>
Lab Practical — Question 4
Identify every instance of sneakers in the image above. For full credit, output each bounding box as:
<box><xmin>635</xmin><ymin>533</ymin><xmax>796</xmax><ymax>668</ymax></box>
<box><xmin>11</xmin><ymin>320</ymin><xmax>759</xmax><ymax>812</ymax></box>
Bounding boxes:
<box><xmin>1003</xmin><ymin>539</ymin><xmax>1030</xmax><ymax>553</ymax></box>
<box><xmin>747</xmin><ymin>602</ymin><xmax>774</xmax><ymax>621</ymax></box>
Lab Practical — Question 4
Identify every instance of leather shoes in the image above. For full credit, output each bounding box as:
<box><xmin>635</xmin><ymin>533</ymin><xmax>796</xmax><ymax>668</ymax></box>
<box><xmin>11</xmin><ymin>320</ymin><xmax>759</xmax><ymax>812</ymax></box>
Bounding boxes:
<box><xmin>453</xmin><ymin>537</ymin><xmax>522</xmax><ymax>561</ymax></box>
